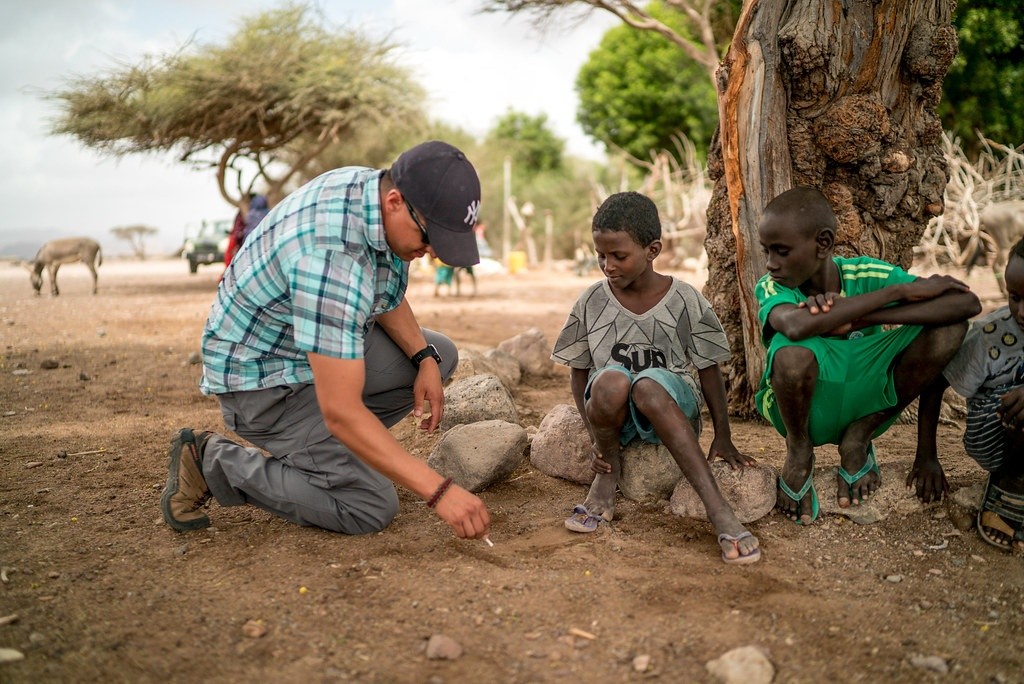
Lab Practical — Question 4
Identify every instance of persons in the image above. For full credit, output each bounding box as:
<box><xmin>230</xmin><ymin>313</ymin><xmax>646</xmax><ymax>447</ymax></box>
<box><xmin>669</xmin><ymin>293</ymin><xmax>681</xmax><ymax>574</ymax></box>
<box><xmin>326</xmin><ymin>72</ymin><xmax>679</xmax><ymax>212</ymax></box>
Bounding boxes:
<box><xmin>754</xmin><ymin>186</ymin><xmax>983</xmax><ymax>524</ymax></box>
<box><xmin>429</xmin><ymin>246</ymin><xmax>478</xmax><ymax>297</ymax></box>
<box><xmin>160</xmin><ymin>140</ymin><xmax>491</xmax><ymax>540</ymax></box>
<box><xmin>550</xmin><ymin>192</ymin><xmax>762</xmax><ymax>565</ymax></box>
<box><xmin>905</xmin><ymin>237</ymin><xmax>1024</xmax><ymax>550</ymax></box>
<box><xmin>217</xmin><ymin>193</ymin><xmax>269</xmax><ymax>272</ymax></box>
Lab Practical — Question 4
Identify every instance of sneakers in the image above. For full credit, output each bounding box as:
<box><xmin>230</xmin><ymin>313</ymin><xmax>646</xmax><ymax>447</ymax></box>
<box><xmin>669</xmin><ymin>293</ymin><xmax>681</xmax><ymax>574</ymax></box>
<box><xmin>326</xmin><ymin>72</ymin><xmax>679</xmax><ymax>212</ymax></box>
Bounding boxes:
<box><xmin>160</xmin><ymin>428</ymin><xmax>216</xmax><ymax>531</ymax></box>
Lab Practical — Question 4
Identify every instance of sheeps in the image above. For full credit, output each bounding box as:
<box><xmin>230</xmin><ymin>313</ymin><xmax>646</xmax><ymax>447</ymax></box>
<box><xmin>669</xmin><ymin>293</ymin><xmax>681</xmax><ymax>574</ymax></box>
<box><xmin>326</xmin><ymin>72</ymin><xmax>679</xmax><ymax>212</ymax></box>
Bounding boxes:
<box><xmin>24</xmin><ymin>237</ymin><xmax>102</xmax><ymax>297</ymax></box>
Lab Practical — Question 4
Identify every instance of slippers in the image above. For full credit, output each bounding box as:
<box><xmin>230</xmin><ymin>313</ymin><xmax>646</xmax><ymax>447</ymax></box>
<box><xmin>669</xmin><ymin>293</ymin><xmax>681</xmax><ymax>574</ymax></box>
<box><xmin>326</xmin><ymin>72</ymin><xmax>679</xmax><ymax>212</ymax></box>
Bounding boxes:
<box><xmin>565</xmin><ymin>504</ymin><xmax>605</xmax><ymax>532</ymax></box>
<box><xmin>978</xmin><ymin>471</ymin><xmax>1024</xmax><ymax>550</ymax></box>
<box><xmin>838</xmin><ymin>440</ymin><xmax>880</xmax><ymax>507</ymax></box>
<box><xmin>717</xmin><ymin>531</ymin><xmax>761</xmax><ymax>565</ymax></box>
<box><xmin>779</xmin><ymin>453</ymin><xmax>818</xmax><ymax>524</ymax></box>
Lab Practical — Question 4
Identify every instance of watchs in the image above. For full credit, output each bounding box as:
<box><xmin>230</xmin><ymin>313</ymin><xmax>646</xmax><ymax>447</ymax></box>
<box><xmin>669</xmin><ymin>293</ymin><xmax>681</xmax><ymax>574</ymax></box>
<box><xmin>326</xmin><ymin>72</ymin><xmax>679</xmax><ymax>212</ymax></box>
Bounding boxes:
<box><xmin>412</xmin><ymin>344</ymin><xmax>442</xmax><ymax>367</ymax></box>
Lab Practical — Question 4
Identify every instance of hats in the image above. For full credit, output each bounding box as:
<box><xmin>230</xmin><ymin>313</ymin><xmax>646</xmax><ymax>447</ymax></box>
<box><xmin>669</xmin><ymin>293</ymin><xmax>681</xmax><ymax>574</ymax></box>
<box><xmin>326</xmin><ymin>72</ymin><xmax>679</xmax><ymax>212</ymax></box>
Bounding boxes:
<box><xmin>391</xmin><ymin>141</ymin><xmax>481</xmax><ymax>267</ymax></box>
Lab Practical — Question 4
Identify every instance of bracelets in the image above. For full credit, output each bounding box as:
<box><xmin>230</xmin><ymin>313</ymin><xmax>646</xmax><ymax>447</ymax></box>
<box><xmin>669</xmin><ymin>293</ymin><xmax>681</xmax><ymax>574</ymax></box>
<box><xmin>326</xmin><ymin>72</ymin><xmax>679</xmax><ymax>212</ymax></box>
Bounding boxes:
<box><xmin>427</xmin><ymin>477</ymin><xmax>455</xmax><ymax>508</ymax></box>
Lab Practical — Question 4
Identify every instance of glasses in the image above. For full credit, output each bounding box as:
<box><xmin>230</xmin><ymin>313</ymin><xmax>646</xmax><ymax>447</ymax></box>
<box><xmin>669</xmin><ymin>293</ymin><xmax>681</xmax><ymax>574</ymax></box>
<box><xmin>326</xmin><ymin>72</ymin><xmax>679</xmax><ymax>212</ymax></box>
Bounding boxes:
<box><xmin>401</xmin><ymin>194</ymin><xmax>430</xmax><ymax>244</ymax></box>
